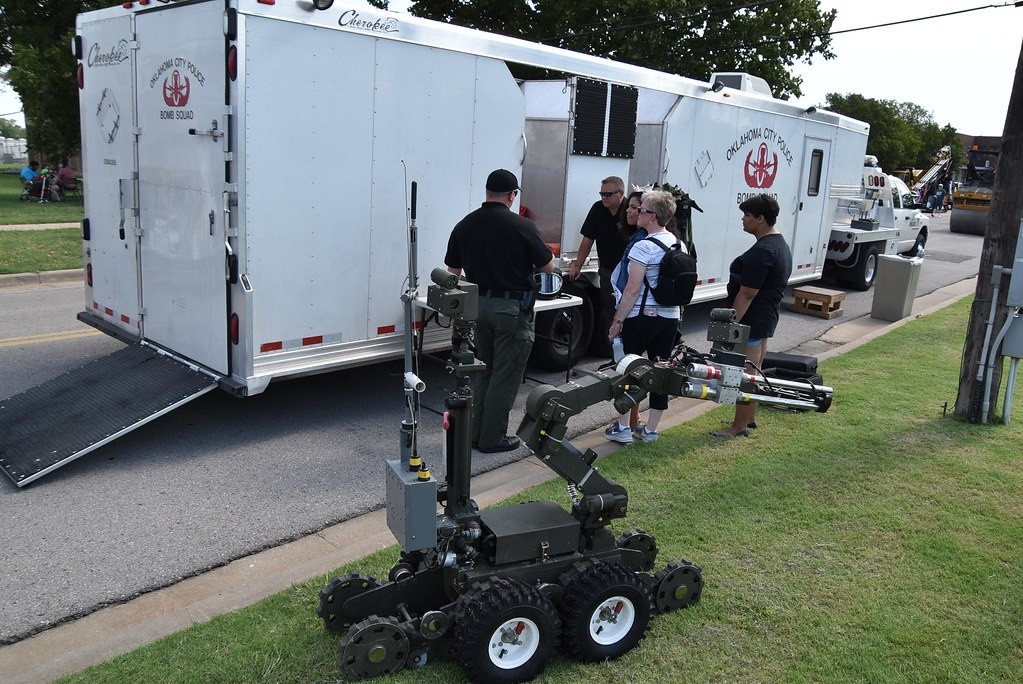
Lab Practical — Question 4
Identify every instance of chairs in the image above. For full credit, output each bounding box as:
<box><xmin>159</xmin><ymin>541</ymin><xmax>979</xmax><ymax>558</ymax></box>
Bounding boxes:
<box><xmin>20</xmin><ymin>177</ymin><xmax>83</xmax><ymax>204</ymax></box>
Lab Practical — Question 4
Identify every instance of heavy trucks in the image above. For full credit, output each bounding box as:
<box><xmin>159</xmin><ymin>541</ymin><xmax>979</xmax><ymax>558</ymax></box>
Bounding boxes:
<box><xmin>0</xmin><ymin>0</ymin><xmax>930</xmax><ymax>483</ymax></box>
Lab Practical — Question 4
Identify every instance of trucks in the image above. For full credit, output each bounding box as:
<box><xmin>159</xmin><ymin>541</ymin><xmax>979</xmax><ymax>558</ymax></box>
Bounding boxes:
<box><xmin>891</xmin><ymin>145</ymin><xmax>954</xmax><ymax>212</ymax></box>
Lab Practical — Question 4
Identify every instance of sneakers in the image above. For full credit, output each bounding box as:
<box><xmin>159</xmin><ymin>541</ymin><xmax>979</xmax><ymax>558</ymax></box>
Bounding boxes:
<box><xmin>604</xmin><ymin>421</ymin><xmax>633</xmax><ymax>442</ymax></box>
<box><xmin>633</xmin><ymin>425</ymin><xmax>658</xmax><ymax>443</ymax></box>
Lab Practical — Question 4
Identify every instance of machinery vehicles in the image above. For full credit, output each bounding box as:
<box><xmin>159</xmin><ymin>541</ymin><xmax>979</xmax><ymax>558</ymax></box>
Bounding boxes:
<box><xmin>949</xmin><ymin>145</ymin><xmax>1001</xmax><ymax>236</ymax></box>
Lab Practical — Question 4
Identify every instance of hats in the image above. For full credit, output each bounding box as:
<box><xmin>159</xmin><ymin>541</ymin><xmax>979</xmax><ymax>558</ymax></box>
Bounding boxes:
<box><xmin>486</xmin><ymin>169</ymin><xmax>523</xmax><ymax>192</ymax></box>
<box><xmin>45</xmin><ymin>173</ymin><xmax>51</xmax><ymax>178</ymax></box>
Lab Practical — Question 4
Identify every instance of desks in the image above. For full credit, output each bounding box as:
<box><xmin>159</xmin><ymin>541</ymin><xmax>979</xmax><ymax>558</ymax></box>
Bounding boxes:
<box><xmin>416</xmin><ymin>291</ymin><xmax>584</xmax><ymax>417</ymax></box>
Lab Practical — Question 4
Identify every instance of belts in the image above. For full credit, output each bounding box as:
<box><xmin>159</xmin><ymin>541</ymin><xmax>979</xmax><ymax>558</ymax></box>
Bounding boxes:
<box><xmin>478</xmin><ymin>289</ymin><xmax>522</xmax><ymax>300</ymax></box>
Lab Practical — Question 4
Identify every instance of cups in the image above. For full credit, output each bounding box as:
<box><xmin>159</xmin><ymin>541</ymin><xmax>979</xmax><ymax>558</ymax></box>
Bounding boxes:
<box><xmin>544</xmin><ymin>243</ymin><xmax>559</xmax><ymax>257</ymax></box>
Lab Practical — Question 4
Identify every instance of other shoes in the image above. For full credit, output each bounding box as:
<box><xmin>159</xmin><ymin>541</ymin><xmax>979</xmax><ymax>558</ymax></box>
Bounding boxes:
<box><xmin>611</xmin><ymin>421</ymin><xmax>642</xmax><ymax>433</ymax></box>
<box><xmin>722</xmin><ymin>421</ymin><xmax>757</xmax><ymax>429</ymax></box>
<box><xmin>710</xmin><ymin>429</ymin><xmax>749</xmax><ymax>437</ymax></box>
<box><xmin>598</xmin><ymin>360</ymin><xmax>614</xmax><ymax>370</ymax></box>
<box><xmin>55</xmin><ymin>199</ymin><xmax>65</xmax><ymax>203</ymax></box>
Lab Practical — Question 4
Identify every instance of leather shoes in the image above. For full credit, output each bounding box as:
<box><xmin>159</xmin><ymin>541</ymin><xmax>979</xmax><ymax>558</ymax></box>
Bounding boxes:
<box><xmin>479</xmin><ymin>435</ymin><xmax>520</xmax><ymax>453</ymax></box>
<box><xmin>471</xmin><ymin>441</ymin><xmax>479</xmax><ymax>449</ymax></box>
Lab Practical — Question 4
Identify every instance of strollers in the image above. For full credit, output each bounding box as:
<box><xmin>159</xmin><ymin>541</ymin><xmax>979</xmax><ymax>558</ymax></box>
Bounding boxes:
<box><xmin>20</xmin><ymin>169</ymin><xmax>54</xmax><ymax>203</ymax></box>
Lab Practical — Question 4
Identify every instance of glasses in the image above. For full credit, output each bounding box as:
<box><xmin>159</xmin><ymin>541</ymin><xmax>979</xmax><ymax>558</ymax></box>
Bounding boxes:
<box><xmin>599</xmin><ymin>191</ymin><xmax>620</xmax><ymax>197</ymax></box>
<box><xmin>512</xmin><ymin>190</ymin><xmax>518</xmax><ymax>196</ymax></box>
<box><xmin>637</xmin><ymin>207</ymin><xmax>658</xmax><ymax>218</ymax></box>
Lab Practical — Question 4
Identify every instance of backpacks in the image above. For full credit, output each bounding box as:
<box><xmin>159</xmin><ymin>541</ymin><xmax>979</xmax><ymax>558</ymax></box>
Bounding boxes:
<box><xmin>637</xmin><ymin>237</ymin><xmax>698</xmax><ymax>331</ymax></box>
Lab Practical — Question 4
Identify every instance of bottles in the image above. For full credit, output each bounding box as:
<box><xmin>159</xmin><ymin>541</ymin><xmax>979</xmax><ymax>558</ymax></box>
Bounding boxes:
<box><xmin>612</xmin><ymin>331</ymin><xmax>625</xmax><ymax>363</ymax></box>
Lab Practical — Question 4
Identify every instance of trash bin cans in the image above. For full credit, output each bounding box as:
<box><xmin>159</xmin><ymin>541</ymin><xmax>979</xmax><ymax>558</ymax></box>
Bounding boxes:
<box><xmin>871</xmin><ymin>254</ymin><xmax>921</xmax><ymax>322</ymax></box>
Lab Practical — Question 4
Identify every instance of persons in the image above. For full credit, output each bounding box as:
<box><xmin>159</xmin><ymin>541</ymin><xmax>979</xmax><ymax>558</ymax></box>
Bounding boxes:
<box><xmin>568</xmin><ymin>175</ymin><xmax>688</xmax><ymax>442</ymax></box>
<box><xmin>709</xmin><ymin>195</ymin><xmax>793</xmax><ymax>438</ymax></box>
<box><xmin>21</xmin><ymin>157</ymin><xmax>77</xmax><ymax>203</ymax></box>
<box><xmin>444</xmin><ymin>168</ymin><xmax>556</xmax><ymax>454</ymax></box>
<box><xmin>931</xmin><ymin>183</ymin><xmax>946</xmax><ymax>217</ymax></box>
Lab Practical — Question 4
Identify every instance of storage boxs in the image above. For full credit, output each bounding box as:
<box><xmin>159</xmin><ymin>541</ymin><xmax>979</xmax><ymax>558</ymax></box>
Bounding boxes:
<box><xmin>790</xmin><ymin>285</ymin><xmax>847</xmax><ymax>320</ymax></box>
